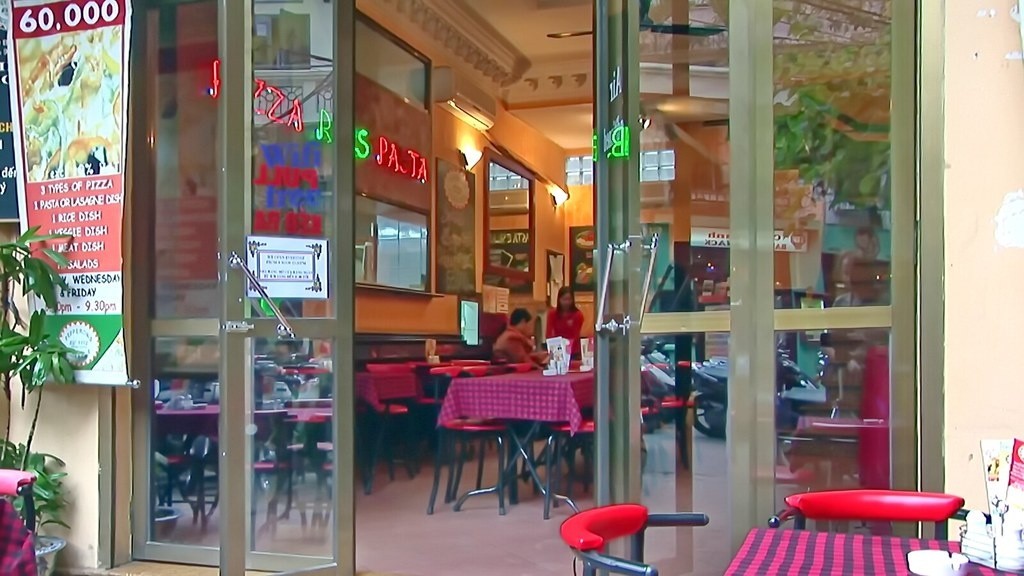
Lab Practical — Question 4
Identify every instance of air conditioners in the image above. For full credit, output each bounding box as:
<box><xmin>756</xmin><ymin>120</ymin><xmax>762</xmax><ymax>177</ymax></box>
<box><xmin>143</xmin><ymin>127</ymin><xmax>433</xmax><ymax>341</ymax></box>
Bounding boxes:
<box><xmin>433</xmin><ymin>64</ymin><xmax>496</xmax><ymax>129</ymax></box>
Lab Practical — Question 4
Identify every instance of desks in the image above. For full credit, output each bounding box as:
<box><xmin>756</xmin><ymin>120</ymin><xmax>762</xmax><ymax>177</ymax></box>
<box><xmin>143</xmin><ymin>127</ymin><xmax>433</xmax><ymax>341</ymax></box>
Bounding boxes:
<box><xmin>722</xmin><ymin>528</ymin><xmax>1024</xmax><ymax>576</ymax></box>
<box><xmin>155</xmin><ymin>406</ymin><xmax>287</xmax><ymax>532</ymax></box>
<box><xmin>435</xmin><ymin>369</ymin><xmax>649</xmax><ymax>520</ymax></box>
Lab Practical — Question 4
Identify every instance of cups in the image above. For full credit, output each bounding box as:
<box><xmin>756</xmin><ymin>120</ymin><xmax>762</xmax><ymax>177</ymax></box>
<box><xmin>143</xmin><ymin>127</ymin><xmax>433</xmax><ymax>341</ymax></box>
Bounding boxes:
<box><xmin>429</xmin><ymin>356</ymin><xmax>439</xmax><ymax>364</ymax></box>
<box><xmin>324</xmin><ymin>361</ymin><xmax>332</xmax><ymax>373</ymax></box>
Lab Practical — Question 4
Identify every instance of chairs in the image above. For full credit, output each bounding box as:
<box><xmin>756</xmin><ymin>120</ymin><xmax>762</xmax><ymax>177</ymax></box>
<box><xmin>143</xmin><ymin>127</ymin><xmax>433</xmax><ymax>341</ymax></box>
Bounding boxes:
<box><xmin>153</xmin><ymin>359</ymin><xmax>710</xmax><ymax>576</ymax></box>
<box><xmin>768</xmin><ymin>489</ymin><xmax>994</xmax><ymax>541</ymax></box>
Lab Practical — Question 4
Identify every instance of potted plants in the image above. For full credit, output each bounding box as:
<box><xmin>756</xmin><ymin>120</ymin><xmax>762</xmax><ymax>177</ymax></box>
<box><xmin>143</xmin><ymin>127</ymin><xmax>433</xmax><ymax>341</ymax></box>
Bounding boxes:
<box><xmin>0</xmin><ymin>229</ymin><xmax>83</xmax><ymax>576</ymax></box>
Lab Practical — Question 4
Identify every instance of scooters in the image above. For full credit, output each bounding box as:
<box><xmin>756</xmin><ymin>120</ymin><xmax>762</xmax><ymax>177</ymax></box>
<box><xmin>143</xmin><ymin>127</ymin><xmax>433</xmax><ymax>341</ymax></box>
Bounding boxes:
<box><xmin>639</xmin><ymin>332</ymin><xmax>820</xmax><ymax>438</ymax></box>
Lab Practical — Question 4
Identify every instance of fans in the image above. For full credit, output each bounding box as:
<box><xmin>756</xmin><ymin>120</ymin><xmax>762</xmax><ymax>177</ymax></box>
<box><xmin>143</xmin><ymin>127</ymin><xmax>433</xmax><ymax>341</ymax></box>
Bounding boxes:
<box><xmin>546</xmin><ymin>0</ymin><xmax>725</xmax><ymax>37</ymax></box>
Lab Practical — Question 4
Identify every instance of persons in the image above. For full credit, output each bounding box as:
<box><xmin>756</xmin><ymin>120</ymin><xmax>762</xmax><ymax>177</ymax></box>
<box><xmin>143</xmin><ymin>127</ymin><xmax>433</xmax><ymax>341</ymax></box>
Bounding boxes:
<box><xmin>491</xmin><ymin>308</ymin><xmax>542</xmax><ymax>370</ymax></box>
<box><xmin>546</xmin><ymin>286</ymin><xmax>584</xmax><ymax>361</ymax></box>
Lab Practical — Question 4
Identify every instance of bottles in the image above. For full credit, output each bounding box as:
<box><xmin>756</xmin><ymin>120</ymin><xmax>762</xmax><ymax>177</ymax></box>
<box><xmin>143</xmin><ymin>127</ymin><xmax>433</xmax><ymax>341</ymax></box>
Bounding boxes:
<box><xmin>184</xmin><ymin>396</ymin><xmax>193</xmax><ymax>410</ymax></box>
<box><xmin>177</xmin><ymin>396</ymin><xmax>184</xmax><ymax>409</ymax></box>
<box><xmin>1001</xmin><ymin>516</ymin><xmax>1024</xmax><ymax>570</ymax></box>
<box><xmin>963</xmin><ymin>509</ymin><xmax>992</xmax><ymax>563</ymax></box>
<box><xmin>550</xmin><ymin>347</ymin><xmax>566</xmax><ymax>374</ymax></box>
<box><xmin>276</xmin><ymin>398</ymin><xmax>281</xmax><ymax>410</ymax></box>
